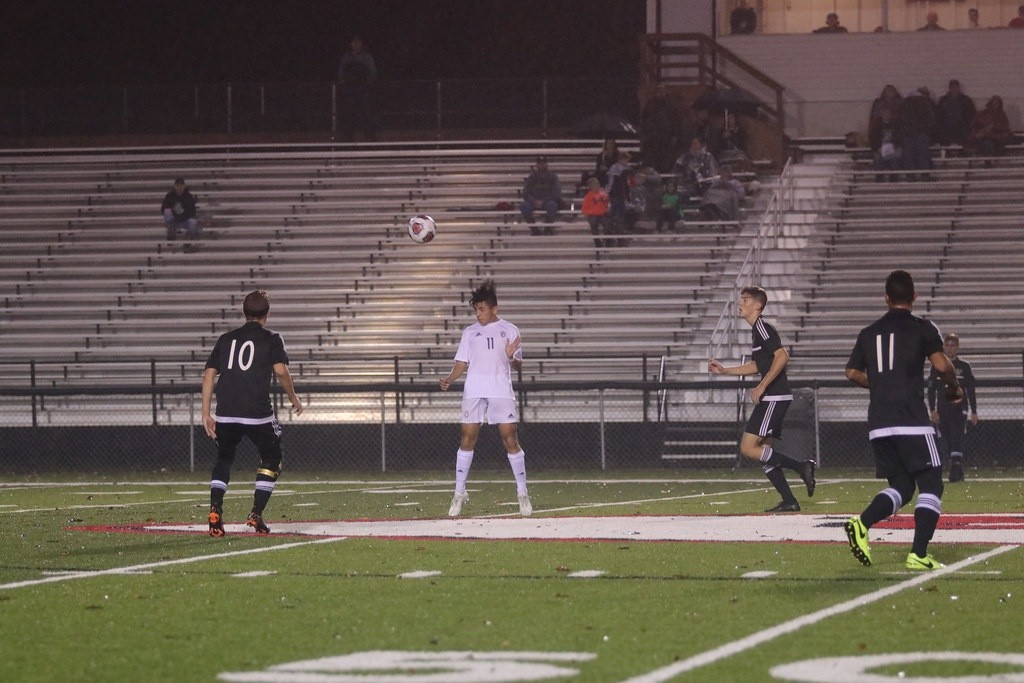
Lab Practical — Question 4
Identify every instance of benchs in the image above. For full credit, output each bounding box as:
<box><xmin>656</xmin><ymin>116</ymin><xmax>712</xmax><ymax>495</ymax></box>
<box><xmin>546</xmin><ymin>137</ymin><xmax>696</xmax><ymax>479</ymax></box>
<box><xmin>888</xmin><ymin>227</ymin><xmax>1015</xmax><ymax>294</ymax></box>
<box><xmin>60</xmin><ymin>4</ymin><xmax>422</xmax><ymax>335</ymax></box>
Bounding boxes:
<box><xmin>0</xmin><ymin>141</ymin><xmax>1024</xmax><ymax>407</ymax></box>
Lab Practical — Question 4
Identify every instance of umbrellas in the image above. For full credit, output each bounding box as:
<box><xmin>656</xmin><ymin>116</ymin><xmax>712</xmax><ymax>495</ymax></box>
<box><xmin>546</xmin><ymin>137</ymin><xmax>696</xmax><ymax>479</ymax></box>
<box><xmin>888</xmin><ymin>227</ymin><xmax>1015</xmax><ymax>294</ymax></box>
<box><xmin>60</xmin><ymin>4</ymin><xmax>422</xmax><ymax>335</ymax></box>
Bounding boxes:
<box><xmin>689</xmin><ymin>86</ymin><xmax>746</xmax><ymax>128</ymax></box>
<box><xmin>564</xmin><ymin>112</ymin><xmax>638</xmax><ymax>151</ymax></box>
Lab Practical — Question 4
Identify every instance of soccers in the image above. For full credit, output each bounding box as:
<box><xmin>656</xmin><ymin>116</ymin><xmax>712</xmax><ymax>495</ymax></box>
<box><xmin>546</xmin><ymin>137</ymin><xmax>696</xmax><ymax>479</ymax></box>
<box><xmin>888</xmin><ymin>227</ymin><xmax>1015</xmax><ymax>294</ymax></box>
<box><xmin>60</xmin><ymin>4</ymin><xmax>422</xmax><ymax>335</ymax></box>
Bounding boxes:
<box><xmin>408</xmin><ymin>214</ymin><xmax>438</xmax><ymax>243</ymax></box>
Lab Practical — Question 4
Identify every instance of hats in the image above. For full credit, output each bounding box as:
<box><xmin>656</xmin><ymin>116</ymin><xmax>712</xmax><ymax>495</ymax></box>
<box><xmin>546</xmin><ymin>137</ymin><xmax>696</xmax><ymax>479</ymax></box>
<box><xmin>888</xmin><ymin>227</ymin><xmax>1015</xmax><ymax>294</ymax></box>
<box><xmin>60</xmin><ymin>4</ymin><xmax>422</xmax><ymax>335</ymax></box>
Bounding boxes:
<box><xmin>536</xmin><ymin>156</ymin><xmax>547</xmax><ymax>163</ymax></box>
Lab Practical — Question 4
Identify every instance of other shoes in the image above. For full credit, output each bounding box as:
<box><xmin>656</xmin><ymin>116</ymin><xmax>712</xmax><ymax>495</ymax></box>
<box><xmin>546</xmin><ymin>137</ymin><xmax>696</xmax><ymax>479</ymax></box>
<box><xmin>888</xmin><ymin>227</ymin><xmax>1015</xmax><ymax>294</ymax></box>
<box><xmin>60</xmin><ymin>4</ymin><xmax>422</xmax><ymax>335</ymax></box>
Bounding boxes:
<box><xmin>948</xmin><ymin>464</ymin><xmax>964</xmax><ymax>482</ymax></box>
<box><xmin>531</xmin><ymin>228</ymin><xmax>541</xmax><ymax>235</ymax></box>
<box><xmin>544</xmin><ymin>228</ymin><xmax>553</xmax><ymax>235</ymax></box>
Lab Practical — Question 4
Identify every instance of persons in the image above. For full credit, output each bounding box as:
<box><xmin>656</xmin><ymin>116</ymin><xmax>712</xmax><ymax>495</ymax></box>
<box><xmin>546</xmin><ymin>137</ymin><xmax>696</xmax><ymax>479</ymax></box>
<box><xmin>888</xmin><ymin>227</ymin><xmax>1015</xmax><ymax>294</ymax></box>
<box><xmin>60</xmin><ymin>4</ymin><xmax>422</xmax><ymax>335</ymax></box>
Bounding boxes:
<box><xmin>162</xmin><ymin>176</ymin><xmax>200</xmax><ymax>249</ymax></box>
<box><xmin>686</xmin><ymin>106</ymin><xmax>748</xmax><ymax>159</ymax></box>
<box><xmin>202</xmin><ymin>292</ymin><xmax>303</xmax><ymax>538</ymax></box>
<box><xmin>844</xmin><ymin>270</ymin><xmax>965</xmax><ymax>573</ymax></box>
<box><xmin>639</xmin><ymin>84</ymin><xmax>683</xmax><ymax>169</ymax></box>
<box><xmin>338</xmin><ymin>31</ymin><xmax>379</xmax><ymax>151</ymax></box>
<box><xmin>521</xmin><ymin>155</ymin><xmax>562</xmax><ymax>236</ymax></box>
<box><xmin>812</xmin><ymin>12</ymin><xmax>847</xmax><ymax>34</ymax></box>
<box><xmin>965</xmin><ymin>9</ymin><xmax>982</xmax><ymax>29</ymax></box>
<box><xmin>730</xmin><ymin>0</ymin><xmax>757</xmax><ymax>34</ymax></box>
<box><xmin>916</xmin><ymin>11</ymin><xmax>947</xmax><ymax>32</ymax></box>
<box><xmin>707</xmin><ymin>286</ymin><xmax>815</xmax><ymax>514</ymax></box>
<box><xmin>1008</xmin><ymin>5</ymin><xmax>1024</xmax><ymax>29</ymax></box>
<box><xmin>926</xmin><ymin>333</ymin><xmax>977</xmax><ymax>484</ymax></box>
<box><xmin>868</xmin><ymin>85</ymin><xmax>937</xmax><ymax>182</ymax></box>
<box><xmin>933</xmin><ymin>80</ymin><xmax>976</xmax><ymax>158</ymax></box>
<box><xmin>441</xmin><ymin>278</ymin><xmax>534</xmax><ymax>516</ymax></box>
<box><xmin>968</xmin><ymin>94</ymin><xmax>1013</xmax><ymax>169</ymax></box>
<box><xmin>578</xmin><ymin>139</ymin><xmax>745</xmax><ymax>252</ymax></box>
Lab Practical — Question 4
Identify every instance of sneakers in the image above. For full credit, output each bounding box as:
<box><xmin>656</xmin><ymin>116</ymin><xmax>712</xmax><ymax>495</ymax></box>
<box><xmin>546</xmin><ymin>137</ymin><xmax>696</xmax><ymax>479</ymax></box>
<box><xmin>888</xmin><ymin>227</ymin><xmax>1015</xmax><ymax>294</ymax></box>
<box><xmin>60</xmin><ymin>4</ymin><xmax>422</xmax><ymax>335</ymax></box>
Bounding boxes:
<box><xmin>799</xmin><ymin>459</ymin><xmax>817</xmax><ymax>497</ymax></box>
<box><xmin>846</xmin><ymin>516</ymin><xmax>873</xmax><ymax>566</ymax></box>
<box><xmin>764</xmin><ymin>498</ymin><xmax>800</xmax><ymax>513</ymax></box>
<box><xmin>905</xmin><ymin>553</ymin><xmax>946</xmax><ymax>571</ymax></box>
<box><xmin>207</xmin><ymin>505</ymin><xmax>226</xmax><ymax>536</ymax></box>
<box><xmin>246</xmin><ymin>513</ymin><xmax>271</xmax><ymax>534</ymax></box>
<box><xmin>517</xmin><ymin>488</ymin><xmax>533</xmax><ymax>516</ymax></box>
<box><xmin>448</xmin><ymin>490</ymin><xmax>470</xmax><ymax>517</ymax></box>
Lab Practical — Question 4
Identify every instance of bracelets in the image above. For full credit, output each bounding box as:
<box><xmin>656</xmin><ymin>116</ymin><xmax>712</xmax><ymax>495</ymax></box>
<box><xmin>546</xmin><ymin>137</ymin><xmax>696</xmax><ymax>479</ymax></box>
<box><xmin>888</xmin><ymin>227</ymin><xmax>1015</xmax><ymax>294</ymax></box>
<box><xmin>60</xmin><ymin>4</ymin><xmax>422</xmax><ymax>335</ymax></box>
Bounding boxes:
<box><xmin>950</xmin><ymin>382</ymin><xmax>960</xmax><ymax>390</ymax></box>
